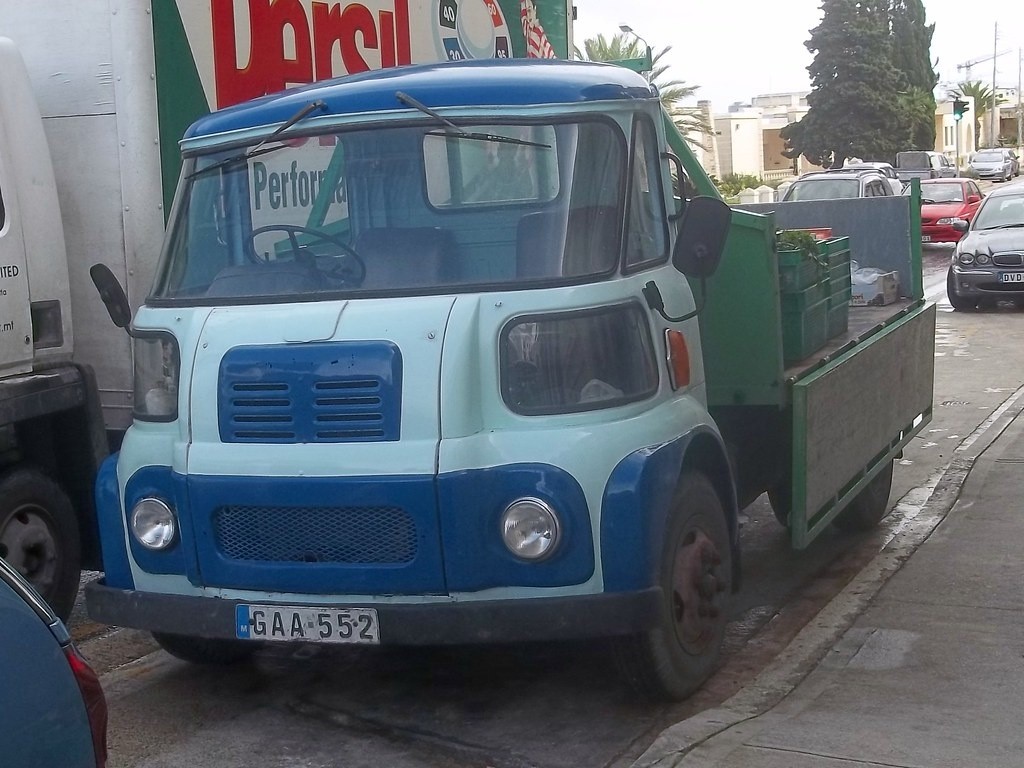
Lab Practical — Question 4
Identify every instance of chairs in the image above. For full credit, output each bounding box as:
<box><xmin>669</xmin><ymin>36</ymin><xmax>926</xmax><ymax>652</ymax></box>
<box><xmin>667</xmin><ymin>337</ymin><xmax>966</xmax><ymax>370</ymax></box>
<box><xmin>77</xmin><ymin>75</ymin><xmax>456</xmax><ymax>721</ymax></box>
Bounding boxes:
<box><xmin>335</xmin><ymin>224</ymin><xmax>453</xmax><ymax>288</ymax></box>
<box><xmin>514</xmin><ymin>203</ymin><xmax>621</xmax><ymax>280</ymax></box>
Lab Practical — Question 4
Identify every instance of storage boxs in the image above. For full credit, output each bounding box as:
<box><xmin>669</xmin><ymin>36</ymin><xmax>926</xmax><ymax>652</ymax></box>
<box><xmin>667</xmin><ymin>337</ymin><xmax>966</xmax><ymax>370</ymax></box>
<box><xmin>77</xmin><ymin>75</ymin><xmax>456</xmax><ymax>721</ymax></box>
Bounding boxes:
<box><xmin>775</xmin><ymin>235</ymin><xmax>852</xmax><ymax>361</ymax></box>
<box><xmin>849</xmin><ymin>269</ymin><xmax>901</xmax><ymax>307</ymax></box>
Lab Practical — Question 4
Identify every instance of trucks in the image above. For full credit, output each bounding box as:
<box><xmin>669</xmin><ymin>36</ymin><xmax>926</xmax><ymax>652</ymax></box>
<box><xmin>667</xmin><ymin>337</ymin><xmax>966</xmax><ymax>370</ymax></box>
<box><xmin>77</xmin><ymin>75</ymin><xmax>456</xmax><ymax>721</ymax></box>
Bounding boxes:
<box><xmin>84</xmin><ymin>55</ymin><xmax>938</xmax><ymax>704</ymax></box>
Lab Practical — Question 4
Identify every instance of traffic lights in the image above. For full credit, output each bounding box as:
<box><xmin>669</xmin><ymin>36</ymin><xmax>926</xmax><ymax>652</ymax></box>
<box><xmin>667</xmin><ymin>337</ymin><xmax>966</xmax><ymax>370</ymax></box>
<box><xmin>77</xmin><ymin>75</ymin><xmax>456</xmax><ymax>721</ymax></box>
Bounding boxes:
<box><xmin>961</xmin><ymin>101</ymin><xmax>969</xmax><ymax>114</ymax></box>
<box><xmin>953</xmin><ymin>101</ymin><xmax>962</xmax><ymax>121</ymax></box>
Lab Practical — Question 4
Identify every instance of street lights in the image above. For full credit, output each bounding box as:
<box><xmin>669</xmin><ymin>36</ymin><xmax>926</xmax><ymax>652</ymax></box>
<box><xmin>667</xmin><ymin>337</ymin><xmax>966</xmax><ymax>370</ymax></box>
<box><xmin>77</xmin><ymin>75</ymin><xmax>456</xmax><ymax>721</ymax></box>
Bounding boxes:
<box><xmin>620</xmin><ymin>26</ymin><xmax>650</xmax><ymax>84</ymax></box>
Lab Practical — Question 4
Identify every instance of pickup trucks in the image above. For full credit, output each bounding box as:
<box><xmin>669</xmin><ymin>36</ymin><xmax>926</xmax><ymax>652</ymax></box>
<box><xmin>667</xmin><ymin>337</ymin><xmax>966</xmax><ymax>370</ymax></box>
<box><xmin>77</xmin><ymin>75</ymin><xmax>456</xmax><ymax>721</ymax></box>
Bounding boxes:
<box><xmin>893</xmin><ymin>150</ymin><xmax>956</xmax><ymax>185</ymax></box>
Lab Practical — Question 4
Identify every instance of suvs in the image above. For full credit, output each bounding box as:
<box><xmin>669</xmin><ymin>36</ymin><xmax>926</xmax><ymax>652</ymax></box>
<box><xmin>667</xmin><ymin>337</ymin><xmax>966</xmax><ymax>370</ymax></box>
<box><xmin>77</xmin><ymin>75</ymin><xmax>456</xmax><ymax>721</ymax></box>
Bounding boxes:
<box><xmin>779</xmin><ymin>168</ymin><xmax>894</xmax><ymax>203</ymax></box>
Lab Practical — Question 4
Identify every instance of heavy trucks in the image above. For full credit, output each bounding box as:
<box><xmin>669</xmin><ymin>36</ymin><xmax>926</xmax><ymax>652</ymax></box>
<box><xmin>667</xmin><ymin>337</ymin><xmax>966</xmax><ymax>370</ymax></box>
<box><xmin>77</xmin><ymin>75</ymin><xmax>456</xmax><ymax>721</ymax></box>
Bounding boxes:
<box><xmin>0</xmin><ymin>0</ymin><xmax>578</xmax><ymax>631</ymax></box>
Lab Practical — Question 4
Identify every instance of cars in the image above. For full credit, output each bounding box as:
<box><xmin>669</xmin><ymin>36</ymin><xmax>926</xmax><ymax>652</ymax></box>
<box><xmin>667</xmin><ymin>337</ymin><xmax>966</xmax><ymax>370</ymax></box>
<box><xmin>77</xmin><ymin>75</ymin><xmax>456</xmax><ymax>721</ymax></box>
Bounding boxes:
<box><xmin>898</xmin><ymin>177</ymin><xmax>986</xmax><ymax>247</ymax></box>
<box><xmin>0</xmin><ymin>565</ymin><xmax>110</xmax><ymax>766</ymax></box>
<box><xmin>969</xmin><ymin>148</ymin><xmax>1021</xmax><ymax>183</ymax></box>
<box><xmin>946</xmin><ymin>182</ymin><xmax>1024</xmax><ymax>309</ymax></box>
<box><xmin>839</xmin><ymin>162</ymin><xmax>899</xmax><ymax>181</ymax></box>
